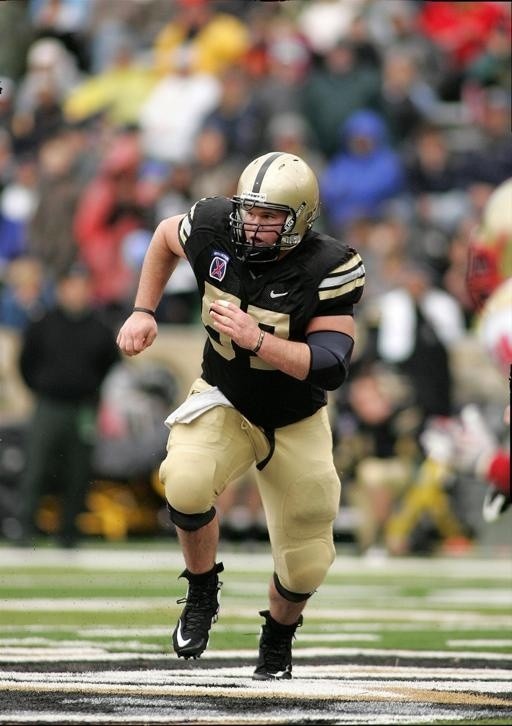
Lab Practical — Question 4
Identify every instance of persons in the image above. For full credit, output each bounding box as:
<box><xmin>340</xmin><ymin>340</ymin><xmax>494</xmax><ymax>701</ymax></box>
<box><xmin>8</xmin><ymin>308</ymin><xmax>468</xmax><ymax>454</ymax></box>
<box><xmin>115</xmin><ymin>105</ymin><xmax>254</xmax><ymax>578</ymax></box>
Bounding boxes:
<box><xmin>0</xmin><ymin>2</ymin><xmax>511</xmax><ymax>557</ymax></box>
<box><xmin>111</xmin><ymin>147</ymin><xmax>366</xmax><ymax>682</ymax></box>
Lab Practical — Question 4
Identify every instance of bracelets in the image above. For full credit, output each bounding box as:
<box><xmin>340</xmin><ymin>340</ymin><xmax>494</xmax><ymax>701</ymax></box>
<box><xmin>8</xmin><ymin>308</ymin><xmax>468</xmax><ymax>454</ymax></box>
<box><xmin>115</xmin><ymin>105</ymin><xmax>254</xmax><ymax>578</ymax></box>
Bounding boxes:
<box><xmin>133</xmin><ymin>308</ymin><xmax>155</xmax><ymax>318</ymax></box>
<box><xmin>252</xmin><ymin>330</ymin><xmax>264</xmax><ymax>352</ymax></box>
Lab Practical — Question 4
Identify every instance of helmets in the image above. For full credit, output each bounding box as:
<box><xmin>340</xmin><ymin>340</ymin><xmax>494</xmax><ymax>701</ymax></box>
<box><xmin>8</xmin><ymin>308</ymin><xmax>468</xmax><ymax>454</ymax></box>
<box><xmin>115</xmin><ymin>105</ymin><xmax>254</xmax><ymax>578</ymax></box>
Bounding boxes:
<box><xmin>228</xmin><ymin>151</ymin><xmax>320</xmax><ymax>269</ymax></box>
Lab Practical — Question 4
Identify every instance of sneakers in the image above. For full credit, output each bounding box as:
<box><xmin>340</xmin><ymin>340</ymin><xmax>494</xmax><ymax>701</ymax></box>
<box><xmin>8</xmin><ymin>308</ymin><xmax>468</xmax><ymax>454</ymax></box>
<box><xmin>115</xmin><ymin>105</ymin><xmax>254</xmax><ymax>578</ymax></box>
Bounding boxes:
<box><xmin>253</xmin><ymin>624</ymin><xmax>293</xmax><ymax>682</ymax></box>
<box><xmin>172</xmin><ymin>577</ymin><xmax>220</xmax><ymax>659</ymax></box>
